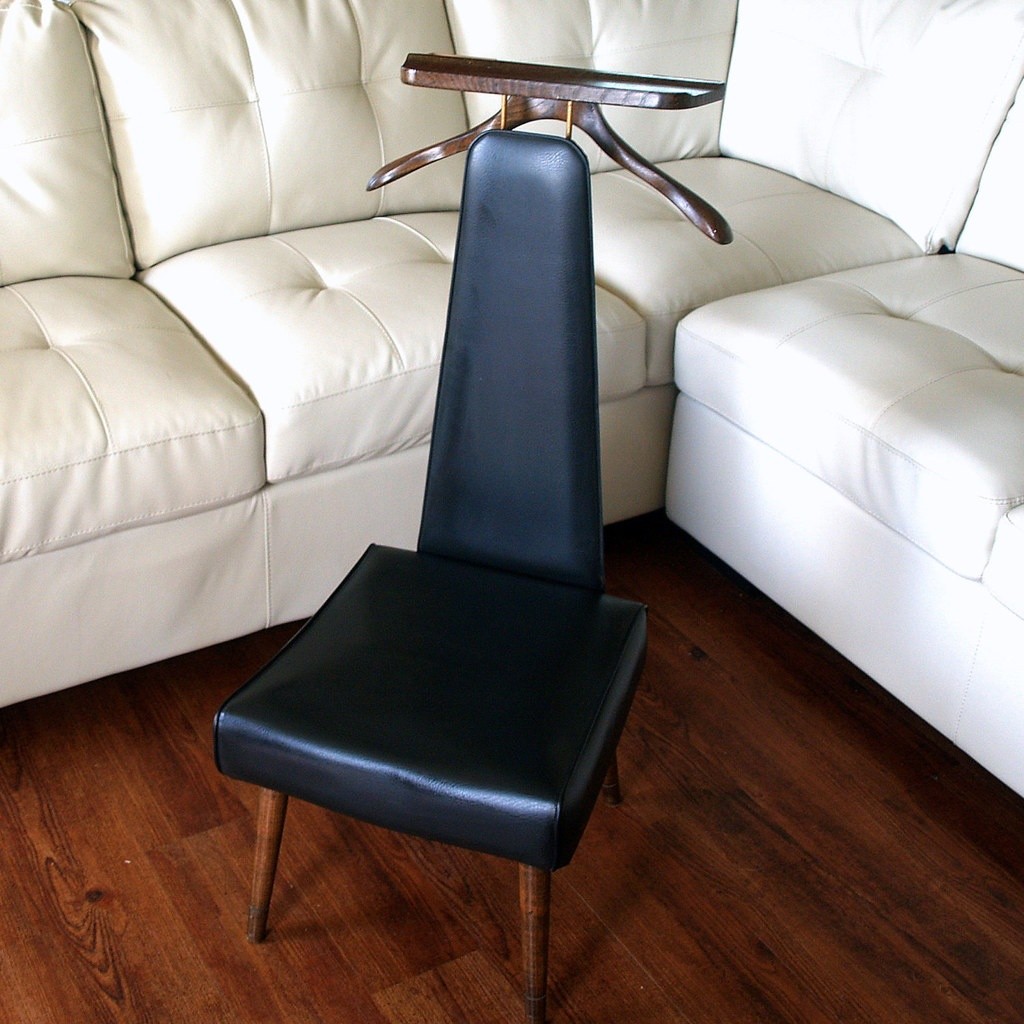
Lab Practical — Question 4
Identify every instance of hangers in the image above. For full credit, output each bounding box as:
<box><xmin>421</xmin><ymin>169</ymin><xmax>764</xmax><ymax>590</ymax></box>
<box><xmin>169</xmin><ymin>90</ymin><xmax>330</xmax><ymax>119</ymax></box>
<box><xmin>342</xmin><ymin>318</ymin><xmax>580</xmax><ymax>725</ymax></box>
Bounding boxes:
<box><xmin>366</xmin><ymin>53</ymin><xmax>733</xmax><ymax>244</ymax></box>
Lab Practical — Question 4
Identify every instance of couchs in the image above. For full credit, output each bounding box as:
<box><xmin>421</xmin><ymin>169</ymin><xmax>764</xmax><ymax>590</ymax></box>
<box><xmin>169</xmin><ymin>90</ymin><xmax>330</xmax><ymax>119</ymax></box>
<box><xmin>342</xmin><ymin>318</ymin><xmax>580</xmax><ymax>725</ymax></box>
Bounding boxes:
<box><xmin>0</xmin><ymin>0</ymin><xmax>1024</xmax><ymax>801</ymax></box>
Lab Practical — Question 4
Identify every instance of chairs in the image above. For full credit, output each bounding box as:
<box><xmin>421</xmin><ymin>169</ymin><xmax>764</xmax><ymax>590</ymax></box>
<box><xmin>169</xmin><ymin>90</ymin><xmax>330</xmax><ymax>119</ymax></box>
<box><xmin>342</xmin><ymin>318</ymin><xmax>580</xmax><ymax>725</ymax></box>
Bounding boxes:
<box><xmin>215</xmin><ymin>131</ymin><xmax>649</xmax><ymax>1024</ymax></box>
